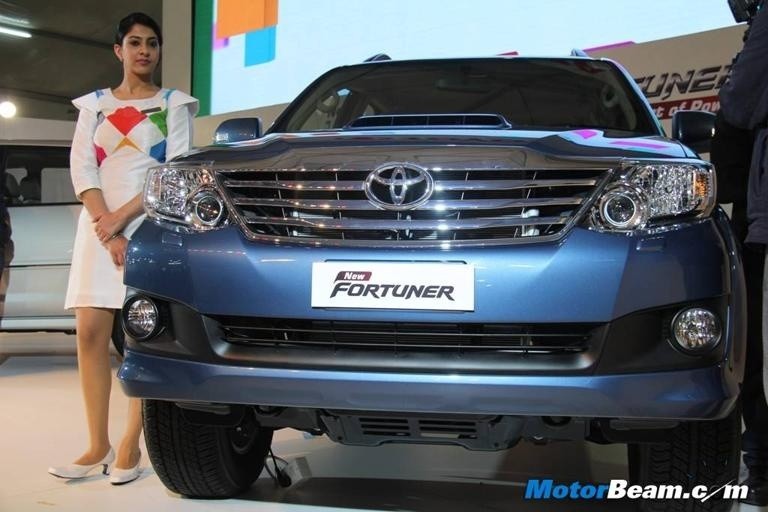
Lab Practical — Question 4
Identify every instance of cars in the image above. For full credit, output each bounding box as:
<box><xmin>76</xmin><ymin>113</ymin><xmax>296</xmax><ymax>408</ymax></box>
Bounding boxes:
<box><xmin>117</xmin><ymin>49</ymin><xmax>748</xmax><ymax>512</ymax></box>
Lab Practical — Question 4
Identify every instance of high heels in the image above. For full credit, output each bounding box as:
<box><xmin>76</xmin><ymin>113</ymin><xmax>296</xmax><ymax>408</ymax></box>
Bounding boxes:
<box><xmin>109</xmin><ymin>448</ymin><xmax>143</xmax><ymax>485</ymax></box>
<box><xmin>48</xmin><ymin>446</ymin><xmax>115</xmax><ymax>480</ymax></box>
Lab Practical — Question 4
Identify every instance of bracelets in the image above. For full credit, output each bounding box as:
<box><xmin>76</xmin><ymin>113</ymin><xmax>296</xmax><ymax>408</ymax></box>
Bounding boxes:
<box><xmin>111</xmin><ymin>232</ymin><xmax>124</xmax><ymax>239</ymax></box>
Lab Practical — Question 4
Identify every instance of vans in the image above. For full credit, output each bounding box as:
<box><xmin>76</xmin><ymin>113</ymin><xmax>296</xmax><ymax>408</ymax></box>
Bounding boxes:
<box><xmin>0</xmin><ymin>117</ymin><xmax>124</xmax><ymax>356</ymax></box>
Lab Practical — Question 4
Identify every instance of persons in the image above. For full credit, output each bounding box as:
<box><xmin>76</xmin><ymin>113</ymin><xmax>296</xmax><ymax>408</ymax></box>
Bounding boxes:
<box><xmin>48</xmin><ymin>12</ymin><xmax>200</xmax><ymax>486</ymax></box>
<box><xmin>717</xmin><ymin>2</ymin><xmax>768</xmax><ymax>505</ymax></box>
